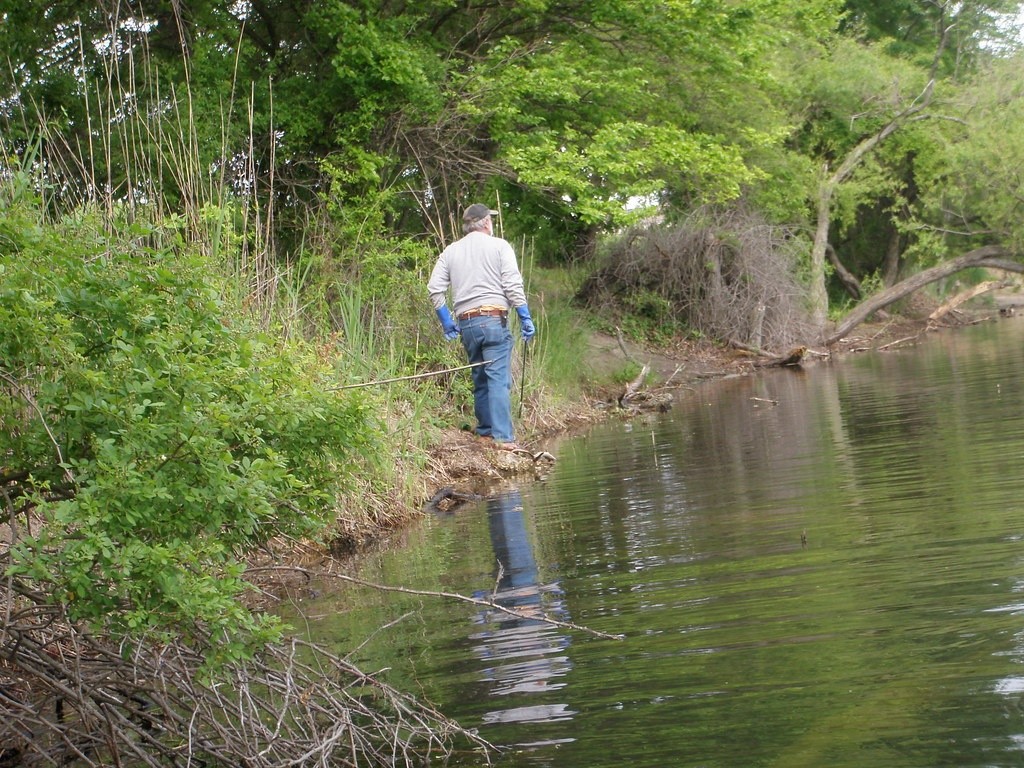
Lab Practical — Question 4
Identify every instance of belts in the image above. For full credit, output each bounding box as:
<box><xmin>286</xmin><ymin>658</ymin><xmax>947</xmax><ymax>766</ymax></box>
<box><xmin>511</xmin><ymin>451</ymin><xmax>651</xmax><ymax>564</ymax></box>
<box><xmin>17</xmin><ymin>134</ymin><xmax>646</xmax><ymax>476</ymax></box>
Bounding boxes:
<box><xmin>459</xmin><ymin>310</ymin><xmax>509</xmax><ymax>320</ymax></box>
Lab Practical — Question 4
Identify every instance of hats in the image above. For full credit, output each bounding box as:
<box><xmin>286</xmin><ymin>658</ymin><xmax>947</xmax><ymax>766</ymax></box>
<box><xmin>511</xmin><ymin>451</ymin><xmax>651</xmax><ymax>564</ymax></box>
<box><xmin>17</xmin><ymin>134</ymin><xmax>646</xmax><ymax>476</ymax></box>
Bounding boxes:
<box><xmin>463</xmin><ymin>203</ymin><xmax>499</xmax><ymax>223</ymax></box>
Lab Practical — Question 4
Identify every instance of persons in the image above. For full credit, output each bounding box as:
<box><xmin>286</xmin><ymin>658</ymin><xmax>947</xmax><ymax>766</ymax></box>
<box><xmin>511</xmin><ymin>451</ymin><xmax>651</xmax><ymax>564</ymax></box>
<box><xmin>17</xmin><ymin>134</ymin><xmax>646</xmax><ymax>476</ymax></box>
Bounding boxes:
<box><xmin>427</xmin><ymin>203</ymin><xmax>535</xmax><ymax>450</ymax></box>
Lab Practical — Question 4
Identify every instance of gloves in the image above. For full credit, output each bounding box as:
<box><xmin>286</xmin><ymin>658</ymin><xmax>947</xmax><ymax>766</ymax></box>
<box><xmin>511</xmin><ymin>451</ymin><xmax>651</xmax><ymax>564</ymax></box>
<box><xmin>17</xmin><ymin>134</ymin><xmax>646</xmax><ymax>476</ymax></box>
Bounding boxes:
<box><xmin>435</xmin><ymin>303</ymin><xmax>461</xmax><ymax>342</ymax></box>
<box><xmin>515</xmin><ymin>303</ymin><xmax>535</xmax><ymax>344</ymax></box>
